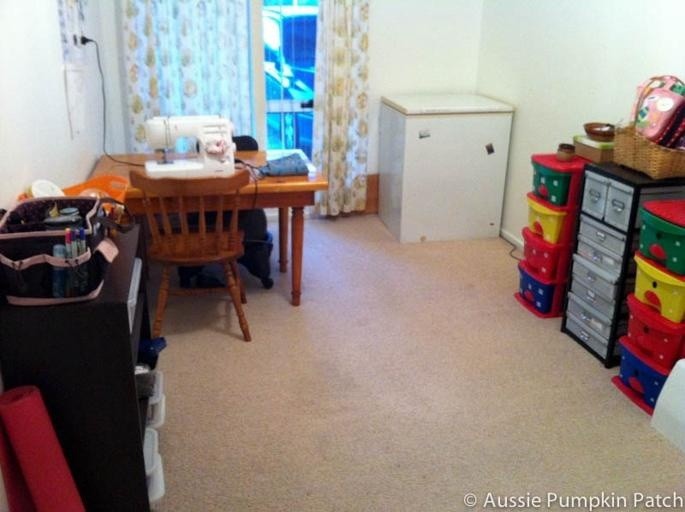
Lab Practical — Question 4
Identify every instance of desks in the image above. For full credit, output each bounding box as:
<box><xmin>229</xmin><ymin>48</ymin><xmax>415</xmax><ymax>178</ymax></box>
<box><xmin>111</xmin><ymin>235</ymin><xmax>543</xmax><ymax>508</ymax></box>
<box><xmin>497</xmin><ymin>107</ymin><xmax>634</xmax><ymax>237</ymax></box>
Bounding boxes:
<box><xmin>89</xmin><ymin>152</ymin><xmax>329</xmax><ymax>306</ymax></box>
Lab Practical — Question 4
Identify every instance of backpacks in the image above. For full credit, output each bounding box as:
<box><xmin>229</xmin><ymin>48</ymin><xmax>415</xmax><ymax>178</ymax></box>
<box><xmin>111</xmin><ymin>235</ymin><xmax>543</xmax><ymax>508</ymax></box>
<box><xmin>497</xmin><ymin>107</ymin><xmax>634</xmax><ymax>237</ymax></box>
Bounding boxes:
<box><xmin>624</xmin><ymin>75</ymin><xmax>685</xmax><ymax>147</ymax></box>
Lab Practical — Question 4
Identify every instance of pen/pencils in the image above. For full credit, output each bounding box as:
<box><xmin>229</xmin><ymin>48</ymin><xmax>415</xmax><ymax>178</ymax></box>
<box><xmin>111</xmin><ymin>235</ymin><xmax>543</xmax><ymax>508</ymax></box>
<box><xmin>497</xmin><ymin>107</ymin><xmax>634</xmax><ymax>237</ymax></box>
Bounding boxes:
<box><xmin>52</xmin><ymin>227</ymin><xmax>88</xmax><ymax>298</ymax></box>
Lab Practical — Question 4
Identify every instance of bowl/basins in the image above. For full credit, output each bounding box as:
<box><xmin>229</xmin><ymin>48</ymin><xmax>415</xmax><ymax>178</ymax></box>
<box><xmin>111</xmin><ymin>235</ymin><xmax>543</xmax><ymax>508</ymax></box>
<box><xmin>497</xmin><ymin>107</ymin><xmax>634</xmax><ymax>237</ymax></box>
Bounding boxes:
<box><xmin>585</xmin><ymin>123</ymin><xmax>615</xmax><ymax>142</ymax></box>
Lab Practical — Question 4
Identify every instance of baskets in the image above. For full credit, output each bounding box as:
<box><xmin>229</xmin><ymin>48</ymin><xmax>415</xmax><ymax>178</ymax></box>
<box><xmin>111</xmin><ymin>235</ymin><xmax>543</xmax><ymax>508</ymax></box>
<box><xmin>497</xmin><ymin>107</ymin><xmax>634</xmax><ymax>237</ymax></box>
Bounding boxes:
<box><xmin>613</xmin><ymin>127</ymin><xmax>684</xmax><ymax>180</ymax></box>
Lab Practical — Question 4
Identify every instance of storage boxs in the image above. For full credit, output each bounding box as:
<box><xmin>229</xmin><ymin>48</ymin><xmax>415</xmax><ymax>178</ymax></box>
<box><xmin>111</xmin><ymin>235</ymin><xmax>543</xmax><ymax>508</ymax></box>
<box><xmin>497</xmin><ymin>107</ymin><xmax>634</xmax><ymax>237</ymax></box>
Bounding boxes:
<box><xmin>514</xmin><ymin>152</ymin><xmax>684</xmax><ymax>415</ymax></box>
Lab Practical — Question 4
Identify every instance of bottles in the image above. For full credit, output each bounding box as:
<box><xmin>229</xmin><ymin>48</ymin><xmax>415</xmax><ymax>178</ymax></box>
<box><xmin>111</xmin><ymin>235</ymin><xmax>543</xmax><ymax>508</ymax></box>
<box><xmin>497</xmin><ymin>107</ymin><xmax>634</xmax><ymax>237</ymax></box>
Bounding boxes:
<box><xmin>556</xmin><ymin>144</ymin><xmax>575</xmax><ymax>163</ymax></box>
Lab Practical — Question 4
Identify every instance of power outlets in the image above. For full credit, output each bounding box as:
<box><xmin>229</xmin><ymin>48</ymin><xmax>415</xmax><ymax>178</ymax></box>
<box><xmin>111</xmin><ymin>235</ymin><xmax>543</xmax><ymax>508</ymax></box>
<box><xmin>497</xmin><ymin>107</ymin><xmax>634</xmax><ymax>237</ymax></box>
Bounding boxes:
<box><xmin>66</xmin><ymin>33</ymin><xmax>89</xmax><ymax>51</ymax></box>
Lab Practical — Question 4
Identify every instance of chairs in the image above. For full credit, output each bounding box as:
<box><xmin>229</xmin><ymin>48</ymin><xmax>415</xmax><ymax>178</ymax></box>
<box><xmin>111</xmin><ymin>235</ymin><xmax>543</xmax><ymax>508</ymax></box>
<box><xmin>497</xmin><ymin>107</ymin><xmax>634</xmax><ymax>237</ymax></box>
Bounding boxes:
<box><xmin>128</xmin><ymin>167</ymin><xmax>253</xmax><ymax>342</ymax></box>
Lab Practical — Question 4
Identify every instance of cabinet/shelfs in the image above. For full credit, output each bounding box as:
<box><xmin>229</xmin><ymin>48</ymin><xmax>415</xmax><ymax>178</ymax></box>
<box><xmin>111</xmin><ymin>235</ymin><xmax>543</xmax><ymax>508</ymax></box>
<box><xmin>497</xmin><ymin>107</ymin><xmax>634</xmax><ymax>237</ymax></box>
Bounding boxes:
<box><xmin>1</xmin><ymin>221</ymin><xmax>157</xmax><ymax>511</ymax></box>
